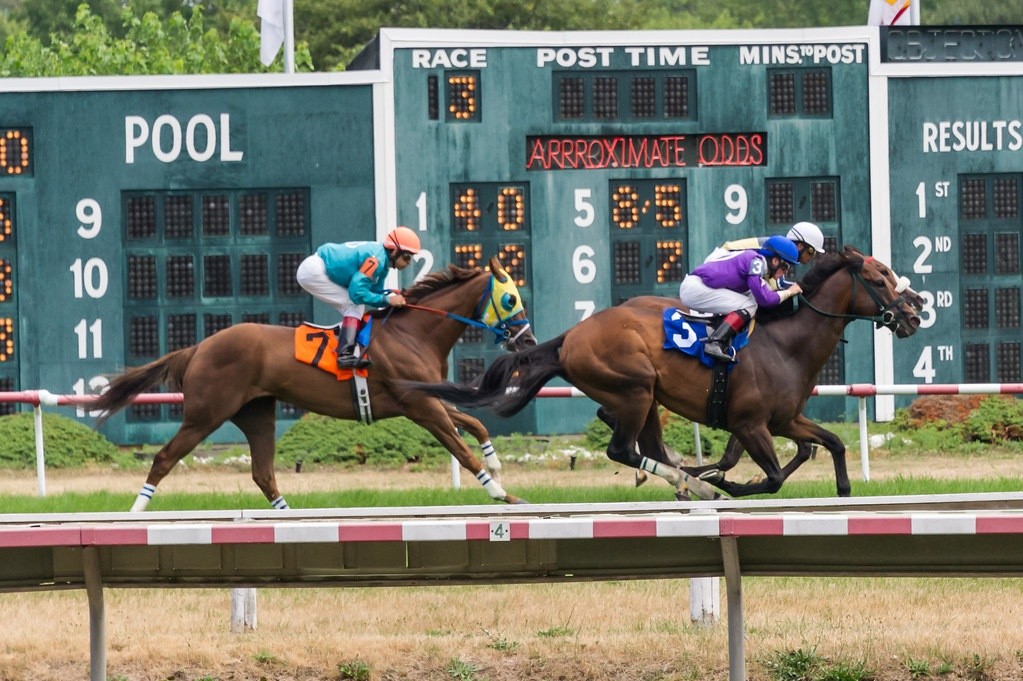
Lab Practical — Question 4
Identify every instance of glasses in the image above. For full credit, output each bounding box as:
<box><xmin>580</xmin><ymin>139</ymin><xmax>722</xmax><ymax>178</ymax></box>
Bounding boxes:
<box><xmin>401</xmin><ymin>253</ymin><xmax>411</xmax><ymax>262</ymax></box>
<box><xmin>804</xmin><ymin>243</ymin><xmax>817</xmax><ymax>257</ymax></box>
<box><xmin>781</xmin><ymin>259</ymin><xmax>793</xmax><ymax>271</ymax></box>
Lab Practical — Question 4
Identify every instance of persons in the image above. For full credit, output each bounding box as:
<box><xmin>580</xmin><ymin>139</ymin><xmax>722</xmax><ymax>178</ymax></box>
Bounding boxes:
<box><xmin>701</xmin><ymin>222</ymin><xmax>825</xmax><ymax>303</ymax></box>
<box><xmin>295</xmin><ymin>226</ymin><xmax>420</xmax><ymax>368</ymax></box>
<box><xmin>680</xmin><ymin>235</ymin><xmax>803</xmax><ymax>362</ymax></box>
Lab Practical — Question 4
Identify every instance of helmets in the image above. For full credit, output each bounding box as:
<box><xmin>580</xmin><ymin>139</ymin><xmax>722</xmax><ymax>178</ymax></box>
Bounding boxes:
<box><xmin>760</xmin><ymin>236</ymin><xmax>801</xmax><ymax>265</ymax></box>
<box><xmin>786</xmin><ymin>222</ymin><xmax>825</xmax><ymax>254</ymax></box>
<box><xmin>384</xmin><ymin>226</ymin><xmax>420</xmax><ymax>254</ymax></box>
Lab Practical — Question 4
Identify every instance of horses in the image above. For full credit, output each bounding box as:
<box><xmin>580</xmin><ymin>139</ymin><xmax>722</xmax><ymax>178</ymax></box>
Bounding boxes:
<box><xmin>389</xmin><ymin>245</ymin><xmax>925</xmax><ymax>500</ymax></box>
<box><xmin>82</xmin><ymin>255</ymin><xmax>539</xmax><ymax>512</ymax></box>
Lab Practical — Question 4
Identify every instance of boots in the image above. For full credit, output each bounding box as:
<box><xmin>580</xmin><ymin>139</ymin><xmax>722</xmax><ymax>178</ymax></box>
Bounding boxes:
<box><xmin>705</xmin><ymin>311</ymin><xmax>746</xmax><ymax>364</ymax></box>
<box><xmin>337</xmin><ymin>316</ymin><xmax>372</xmax><ymax>367</ymax></box>
